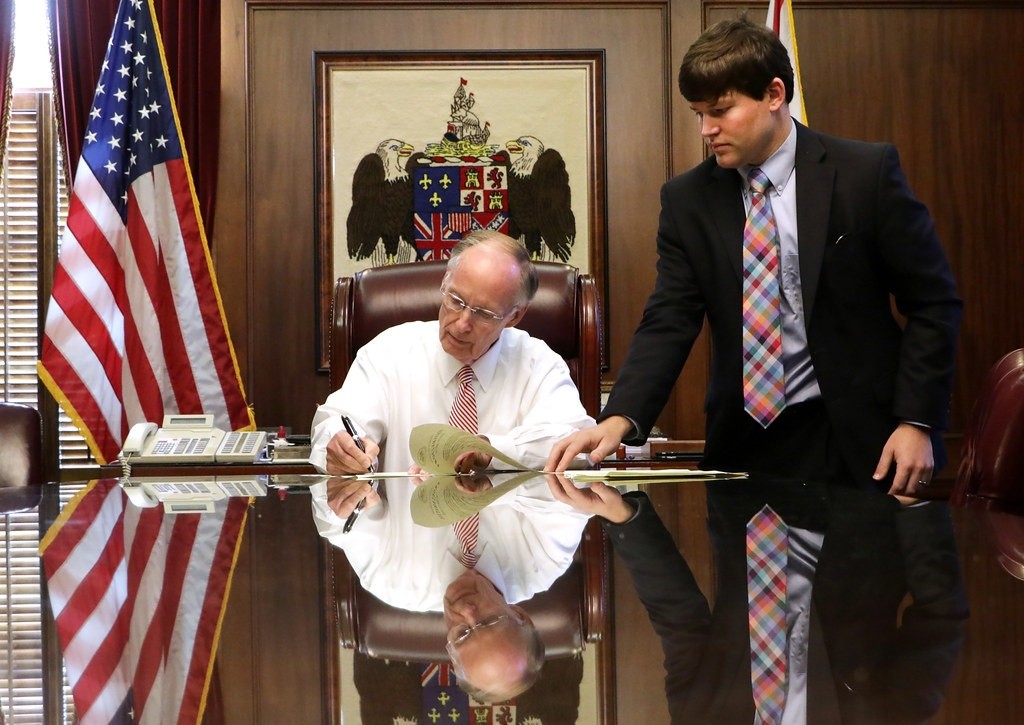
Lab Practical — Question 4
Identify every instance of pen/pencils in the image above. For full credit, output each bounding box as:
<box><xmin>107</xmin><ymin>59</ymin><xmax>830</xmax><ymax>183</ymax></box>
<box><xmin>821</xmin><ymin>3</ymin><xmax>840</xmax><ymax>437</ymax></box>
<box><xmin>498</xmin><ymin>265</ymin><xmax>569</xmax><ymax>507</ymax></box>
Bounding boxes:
<box><xmin>835</xmin><ymin>233</ymin><xmax>846</xmax><ymax>245</ymax></box>
<box><xmin>340</xmin><ymin>479</ymin><xmax>376</xmax><ymax>535</ymax></box>
<box><xmin>339</xmin><ymin>414</ymin><xmax>375</xmax><ymax>475</ymax></box>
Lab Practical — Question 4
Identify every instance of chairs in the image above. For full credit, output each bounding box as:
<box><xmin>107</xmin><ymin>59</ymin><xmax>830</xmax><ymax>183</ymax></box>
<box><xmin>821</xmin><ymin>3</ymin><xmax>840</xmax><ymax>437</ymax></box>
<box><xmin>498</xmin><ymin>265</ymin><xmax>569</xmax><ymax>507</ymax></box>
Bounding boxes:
<box><xmin>326</xmin><ymin>258</ymin><xmax>604</xmax><ymax>427</ymax></box>
<box><xmin>327</xmin><ymin>520</ymin><xmax>606</xmax><ymax>664</ymax></box>
<box><xmin>948</xmin><ymin>496</ymin><xmax>1024</xmax><ymax>583</ymax></box>
<box><xmin>948</xmin><ymin>348</ymin><xmax>1024</xmax><ymax>495</ymax></box>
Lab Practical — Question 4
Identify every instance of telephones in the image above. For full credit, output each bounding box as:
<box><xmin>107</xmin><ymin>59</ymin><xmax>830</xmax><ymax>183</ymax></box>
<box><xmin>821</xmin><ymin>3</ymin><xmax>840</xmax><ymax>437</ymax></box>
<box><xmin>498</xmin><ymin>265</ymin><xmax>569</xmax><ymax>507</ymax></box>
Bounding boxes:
<box><xmin>118</xmin><ymin>476</ymin><xmax>226</xmax><ymax>515</ymax></box>
<box><xmin>116</xmin><ymin>413</ymin><xmax>226</xmax><ymax>464</ymax></box>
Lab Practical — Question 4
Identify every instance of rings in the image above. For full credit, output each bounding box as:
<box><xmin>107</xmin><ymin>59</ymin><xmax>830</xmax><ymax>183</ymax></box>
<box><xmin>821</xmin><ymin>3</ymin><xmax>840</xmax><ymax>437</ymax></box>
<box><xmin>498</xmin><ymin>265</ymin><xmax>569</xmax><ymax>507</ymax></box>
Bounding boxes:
<box><xmin>919</xmin><ymin>480</ymin><xmax>927</xmax><ymax>485</ymax></box>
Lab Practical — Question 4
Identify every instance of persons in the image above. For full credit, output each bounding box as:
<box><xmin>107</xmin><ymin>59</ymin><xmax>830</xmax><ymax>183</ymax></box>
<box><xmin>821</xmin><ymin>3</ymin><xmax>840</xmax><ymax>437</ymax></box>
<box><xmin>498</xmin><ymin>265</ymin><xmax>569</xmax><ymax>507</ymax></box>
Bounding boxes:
<box><xmin>307</xmin><ymin>478</ymin><xmax>602</xmax><ymax>706</ymax></box>
<box><xmin>307</xmin><ymin>229</ymin><xmax>601</xmax><ymax>475</ymax></box>
<box><xmin>548</xmin><ymin>477</ymin><xmax>969</xmax><ymax>725</ymax></box>
<box><xmin>542</xmin><ymin>16</ymin><xmax>966</xmax><ymax>497</ymax></box>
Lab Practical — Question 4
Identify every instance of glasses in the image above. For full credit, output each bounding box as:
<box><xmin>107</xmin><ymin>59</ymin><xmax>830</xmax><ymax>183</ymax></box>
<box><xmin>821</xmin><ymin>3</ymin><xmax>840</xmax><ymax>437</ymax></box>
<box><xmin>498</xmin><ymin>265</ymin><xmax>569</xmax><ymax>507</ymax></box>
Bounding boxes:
<box><xmin>445</xmin><ymin>608</ymin><xmax>522</xmax><ymax>665</ymax></box>
<box><xmin>442</xmin><ymin>289</ymin><xmax>517</xmax><ymax>326</ymax></box>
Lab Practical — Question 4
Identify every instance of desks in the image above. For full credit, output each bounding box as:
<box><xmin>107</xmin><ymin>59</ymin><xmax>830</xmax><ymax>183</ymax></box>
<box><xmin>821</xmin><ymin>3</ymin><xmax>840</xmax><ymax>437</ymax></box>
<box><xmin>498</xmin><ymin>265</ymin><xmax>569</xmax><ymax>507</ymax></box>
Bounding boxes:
<box><xmin>0</xmin><ymin>467</ymin><xmax>1024</xmax><ymax>725</ymax></box>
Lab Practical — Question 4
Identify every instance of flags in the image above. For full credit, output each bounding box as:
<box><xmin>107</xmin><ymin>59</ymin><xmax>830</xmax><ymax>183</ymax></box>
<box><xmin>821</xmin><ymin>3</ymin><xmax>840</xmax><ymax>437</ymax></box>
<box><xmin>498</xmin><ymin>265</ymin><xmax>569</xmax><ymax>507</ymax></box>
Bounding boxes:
<box><xmin>39</xmin><ymin>478</ymin><xmax>256</xmax><ymax>725</ymax></box>
<box><xmin>38</xmin><ymin>0</ymin><xmax>257</xmax><ymax>465</ymax></box>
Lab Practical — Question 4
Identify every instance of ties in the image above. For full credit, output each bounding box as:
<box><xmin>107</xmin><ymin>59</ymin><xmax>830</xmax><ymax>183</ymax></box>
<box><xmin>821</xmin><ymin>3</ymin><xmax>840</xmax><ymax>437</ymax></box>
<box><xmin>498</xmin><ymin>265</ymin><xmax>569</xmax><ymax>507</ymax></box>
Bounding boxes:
<box><xmin>743</xmin><ymin>168</ymin><xmax>787</xmax><ymax>429</ymax></box>
<box><xmin>448</xmin><ymin>366</ymin><xmax>479</xmax><ymax>436</ymax></box>
<box><xmin>452</xmin><ymin>512</ymin><xmax>482</xmax><ymax>569</ymax></box>
<box><xmin>746</xmin><ymin>503</ymin><xmax>788</xmax><ymax>725</ymax></box>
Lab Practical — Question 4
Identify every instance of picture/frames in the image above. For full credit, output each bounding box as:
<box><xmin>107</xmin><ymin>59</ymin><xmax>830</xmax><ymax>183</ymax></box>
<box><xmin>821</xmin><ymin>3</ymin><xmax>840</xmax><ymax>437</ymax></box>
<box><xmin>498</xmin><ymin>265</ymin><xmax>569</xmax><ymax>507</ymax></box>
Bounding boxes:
<box><xmin>312</xmin><ymin>51</ymin><xmax>606</xmax><ymax>374</ymax></box>
<box><xmin>319</xmin><ymin>523</ymin><xmax>617</xmax><ymax>725</ymax></box>
